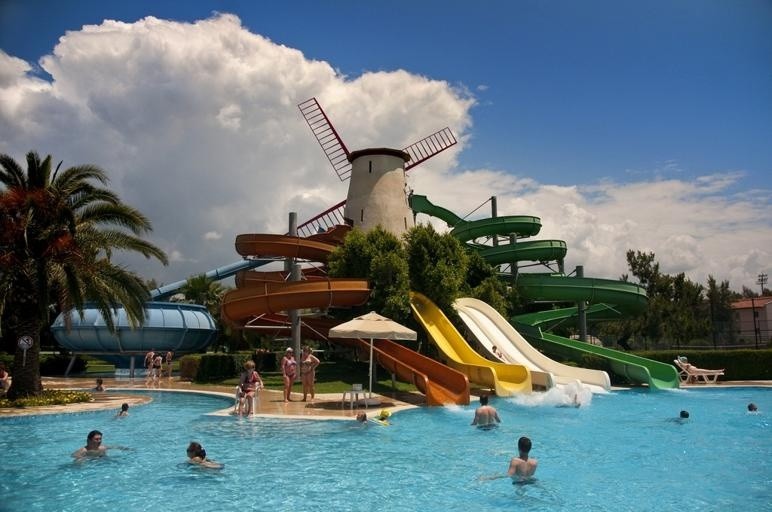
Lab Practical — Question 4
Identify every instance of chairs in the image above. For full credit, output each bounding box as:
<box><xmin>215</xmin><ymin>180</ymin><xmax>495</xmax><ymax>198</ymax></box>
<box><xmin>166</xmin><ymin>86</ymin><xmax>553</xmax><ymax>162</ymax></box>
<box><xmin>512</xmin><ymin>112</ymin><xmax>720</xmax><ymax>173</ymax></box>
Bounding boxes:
<box><xmin>673</xmin><ymin>359</ymin><xmax>725</xmax><ymax>383</ymax></box>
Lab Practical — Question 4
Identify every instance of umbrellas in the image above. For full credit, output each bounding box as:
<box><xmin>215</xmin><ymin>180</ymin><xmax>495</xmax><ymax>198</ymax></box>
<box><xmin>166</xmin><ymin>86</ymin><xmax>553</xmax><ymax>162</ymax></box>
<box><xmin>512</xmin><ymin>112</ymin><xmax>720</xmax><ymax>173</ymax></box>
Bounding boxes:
<box><xmin>328</xmin><ymin>310</ymin><xmax>419</xmax><ymax>399</ymax></box>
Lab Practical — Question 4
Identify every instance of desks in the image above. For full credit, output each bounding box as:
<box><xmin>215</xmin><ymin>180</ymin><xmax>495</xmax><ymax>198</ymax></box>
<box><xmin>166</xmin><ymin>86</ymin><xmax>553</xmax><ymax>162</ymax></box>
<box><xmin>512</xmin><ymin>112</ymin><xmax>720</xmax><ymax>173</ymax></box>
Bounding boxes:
<box><xmin>341</xmin><ymin>390</ymin><xmax>368</xmax><ymax>410</ymax></box>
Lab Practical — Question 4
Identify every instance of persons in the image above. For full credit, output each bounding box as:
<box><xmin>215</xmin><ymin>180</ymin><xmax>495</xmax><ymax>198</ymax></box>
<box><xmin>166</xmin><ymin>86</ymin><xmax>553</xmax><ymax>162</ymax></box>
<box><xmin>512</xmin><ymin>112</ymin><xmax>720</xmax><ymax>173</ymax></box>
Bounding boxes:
<box><xmin>679</xmin><ymin>410</ymin><xmax>690</xmax><ymax>419</ymax></box>
<box><xmin>478</xmin><ymin>436</ymin><xmax>539</xmax><ymax>486</ymax></box>
<box><xmin>280</xmin><ymin>346</ymin><xmax>296</xmax><ymax>403</ymax></box>
<box><xmin>492</xmin><ymin>345</ymin><xmax>504</xmax><ymax>358</ymax></box>
<box><xmin>0</xmin><ymin>364</ymin><xmax>10</xmax><ymax>388</ymax></box>
<box><xmin>299</xmin><ymin>345</ymin><xmax>320</xmax><ymax>402</ymax></box>
<box><xmin>356</xmin><ymin>410</ymin><xmax>368</xmax><ymax>425</ymax></box>
<box><xmin>95</xmin><ymin>379</ymin><xmax>105</xmax><ymax>392</ymax></box>
<box><xmin>117</xmin><ymin>402</ymin><xmax>130</xmax><ymax>417</ymax></box>
<box><xmin>70</xmin><ymin>430</ymin><xmax>107</xmax><ymax>469</ymax></box>
<box><xmin>470</xmin><ymin>393</ymin><xmax>501</xmax><ymax>431</ymax></box>
<box><xmin>186</xmin><ymin>441</ymin><xmax>225</xmax><ymax>479</ymax></box>
<box><xmin>144</xmin><ymin>376</ymin><xmax>160</xmax><ymax>389</ymax></box>
<box><xmin>238</xmin><ymin>360</ymin><xmax>264</xmax><ymax>422</ymax></box>
<box><xmin>748</xmin><ymin>403</ymin><xmax>757</xmax><ymax>412</ymax></box>
<box><xmin>143</xmin><ymin>348</ymin><xmax>175</xmax><ymax>380</ymax></box>
